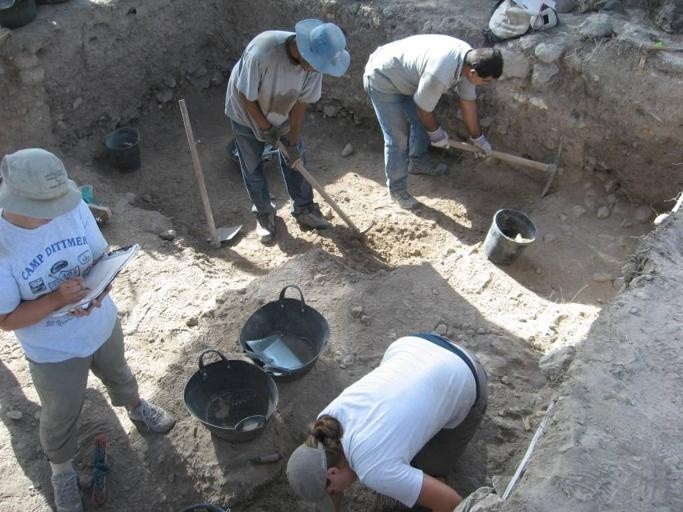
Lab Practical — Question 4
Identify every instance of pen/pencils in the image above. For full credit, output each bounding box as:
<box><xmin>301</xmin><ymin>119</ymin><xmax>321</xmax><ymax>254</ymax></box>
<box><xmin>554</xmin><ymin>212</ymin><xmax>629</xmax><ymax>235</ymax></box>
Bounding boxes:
<box><xmin>48</xmin><ymin>274</ymin><xmax>92</xmax><ymax>290</ymax></box>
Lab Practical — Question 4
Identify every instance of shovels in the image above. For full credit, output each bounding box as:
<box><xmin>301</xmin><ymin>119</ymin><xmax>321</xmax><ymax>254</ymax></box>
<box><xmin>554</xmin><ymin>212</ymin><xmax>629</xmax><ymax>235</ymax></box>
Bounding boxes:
<box><xmin>243</xmin><ymin>335</ymin><xmax>304</xmax><ymax>376</ymax></box>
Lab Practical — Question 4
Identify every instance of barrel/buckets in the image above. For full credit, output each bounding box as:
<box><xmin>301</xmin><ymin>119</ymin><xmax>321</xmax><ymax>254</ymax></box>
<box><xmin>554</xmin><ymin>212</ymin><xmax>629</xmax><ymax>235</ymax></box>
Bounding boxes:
<box><xmin>102</xmin><ymin>126</ymin><xmax>142</xmax><ymax>171</ymax></box>
<box><xmin>483</xmin><ymin>208</ymin><xmax>537</xmax><ymax>266</ymax></box>
<box><xmin>181</xmin><ymin>350</ymin><xmax>279</xmax><ymax>442</ymax></box>
<box><xmin>225</xmin><ymin>138</ymin><xmax>246</xmax><ymax>168</ymax></box>
<box><xmin>0</xmin><ymin>0</ymin><xmax>36</xmax><ymax>29</ymax></box>
<box><xmin>240</xmin><ymin>285</ymin><xmax>330</xmax><ymax>378</ymax></box>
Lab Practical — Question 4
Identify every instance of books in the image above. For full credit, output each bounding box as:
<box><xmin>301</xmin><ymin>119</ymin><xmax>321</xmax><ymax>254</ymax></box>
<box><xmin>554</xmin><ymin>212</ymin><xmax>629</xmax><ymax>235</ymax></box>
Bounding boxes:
<box><xmin>52</xmin><ymin>243</ymin><xmax>141</xmax><ymax>318</ymax></box>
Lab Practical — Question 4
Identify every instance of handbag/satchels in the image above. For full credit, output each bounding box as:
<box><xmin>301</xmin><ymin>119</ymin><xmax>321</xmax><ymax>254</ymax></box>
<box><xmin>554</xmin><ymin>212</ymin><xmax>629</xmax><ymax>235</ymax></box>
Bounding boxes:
<box><xmin>488</xmin><ymin>0</ymin><xmax>558</xmax><ymax>41</ymax></box>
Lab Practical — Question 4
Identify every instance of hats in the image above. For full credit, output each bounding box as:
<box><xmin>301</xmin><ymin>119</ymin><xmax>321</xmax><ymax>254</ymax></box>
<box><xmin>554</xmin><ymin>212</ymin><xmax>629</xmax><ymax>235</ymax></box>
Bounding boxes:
<box><xmin>0</xmin><ymin>147</ymin><xmax>83</xmax><ymax>219</ymax></box>
<box><xmin>286</xmin><ymin>439</ymin><xmax>335</xmax><ymax>511</ymax></box>
<box><xmin>294</xmin><ymin>19</ymin><xmax>351</xmax><ymax>77</ymax></box>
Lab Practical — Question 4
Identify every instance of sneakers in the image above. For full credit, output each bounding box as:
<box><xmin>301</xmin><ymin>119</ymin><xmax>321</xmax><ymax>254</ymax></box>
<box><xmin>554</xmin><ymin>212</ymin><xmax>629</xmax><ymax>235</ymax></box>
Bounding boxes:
<box><xmin>51</xmin><ymin>471</ymin><xmax>84</xmax><ymax>512</ymax></box>
<box><xmin>408</xmin><ymin>160</ymin><xmax>448</xmax><ymax>176</ymax></box>
<box><xmin>257</xmin><ymin>216</ymin><xmax>275</xmax><ymax>244</ymax></box>
<box><xmin>390</xmin><ymin>189</ymin><xmax>419</xmax><ymax>210</ymax></box>
<box><xmin>128</xmin><ymin>398</ymin><xmax>176</xmax><ymax>434</ymax></box>
<box><xmin>295</xmin><ymin>210</ymin><xmax>329</xmax><ymax>229</ymax></box>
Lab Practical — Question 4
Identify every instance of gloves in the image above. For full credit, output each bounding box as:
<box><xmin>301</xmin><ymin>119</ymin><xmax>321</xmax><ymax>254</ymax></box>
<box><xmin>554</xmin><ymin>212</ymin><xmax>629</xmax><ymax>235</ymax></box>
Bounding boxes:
<box><xmin>286</xmin><ymin>145</ymin><xmax>303</xmax><ymax>171</ymax></box>
<box><xmin>427</xmin><ymin>125</ymin><xmax>450</xmax><ymax>149</ymax></box>
<box><xmin>259</xmin><ymin>124</ymin><xmax>281</xmax><ymax>147</ymax></box>
<box><xmin>469</xmin><ymin>135</ymin><xmax>491</xmax><ymax>160</ymax></box>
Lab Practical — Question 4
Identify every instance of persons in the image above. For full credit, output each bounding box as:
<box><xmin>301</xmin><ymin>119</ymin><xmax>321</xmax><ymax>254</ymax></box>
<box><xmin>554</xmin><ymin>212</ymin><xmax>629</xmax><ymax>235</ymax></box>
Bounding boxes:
<box><xmin>286</xmin><ymin>333</ymin><xmax>487</xmax><ymax>512</ymax></box>
<box><xmin>363</xmin><ymin>33</ymin><xmax>503</xmax><ymax>209</ymax></box>
<box><xmin>0</xmin><ymin>148</ymin><xmax>175</xmax><ymax>512</ymax></box>
<box><xmin>224</xmin><ymin>19</ymin><xmax>350</xmax><ymax>243</ymax></box>
